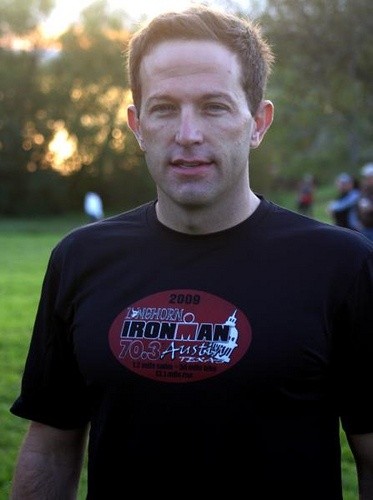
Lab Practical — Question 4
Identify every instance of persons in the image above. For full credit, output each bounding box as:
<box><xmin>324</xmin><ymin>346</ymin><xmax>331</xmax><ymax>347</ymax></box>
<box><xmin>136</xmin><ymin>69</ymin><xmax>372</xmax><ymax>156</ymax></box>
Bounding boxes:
<box><xmin>8</xmin><ymin>8</ymin><xmax>373</xmax><ymax>500</ymax></box>
<box><xmin>296</xmin><ymin>161</ymin><xmax>373</xmax><ymax>243</ymax></box>
